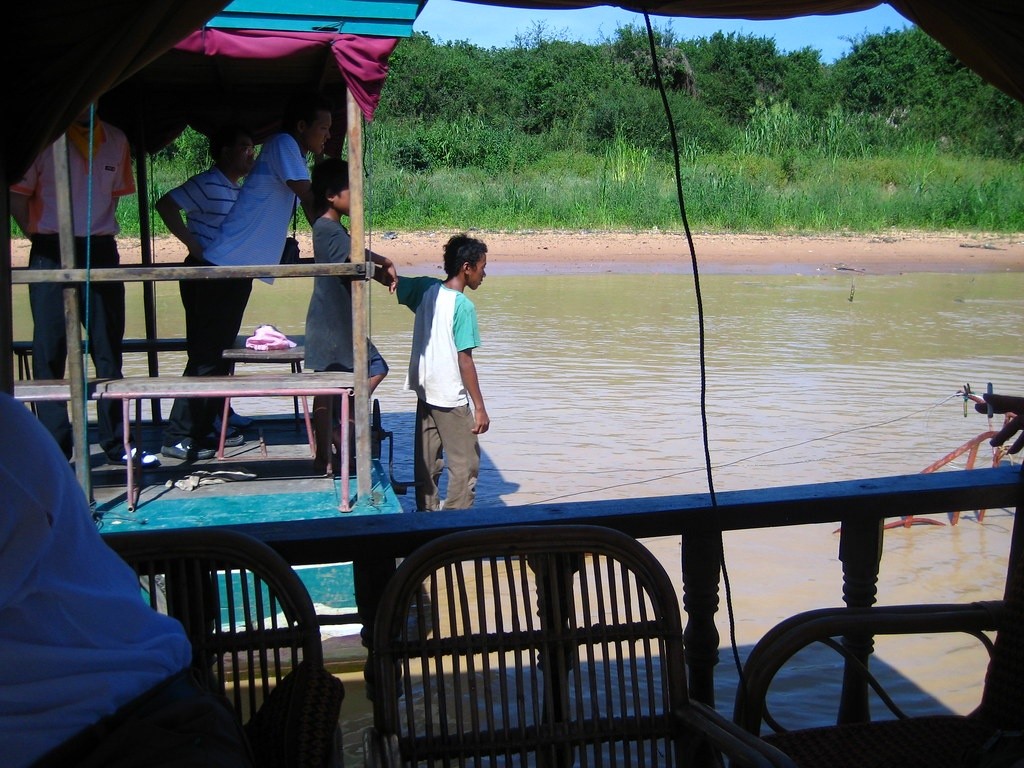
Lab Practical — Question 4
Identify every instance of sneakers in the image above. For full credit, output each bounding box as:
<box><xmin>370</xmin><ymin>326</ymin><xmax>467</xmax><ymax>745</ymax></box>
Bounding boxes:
<box><xmin>161</xmin><ymin>438</ymin><xmax>216</xmax><ymax>459</ymax></box>
<box><xmin>206</xmin><ymin>428</ymin><xmax>245</xmax><ymax>446</ymax></box>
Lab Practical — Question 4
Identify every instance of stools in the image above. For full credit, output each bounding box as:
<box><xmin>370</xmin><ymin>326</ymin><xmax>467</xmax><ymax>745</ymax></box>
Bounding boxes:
<box><xmin>218</xmin><ymin>346</ymin><xmax>318</xmax><ymax>461</ymax></box>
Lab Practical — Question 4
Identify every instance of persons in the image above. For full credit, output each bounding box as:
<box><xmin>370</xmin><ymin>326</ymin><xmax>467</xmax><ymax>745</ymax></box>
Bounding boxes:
<box><xmin>10</xmin><ymin>96</ymin><xmax>159</xmax><ymax>472</ymax></box>
<box><xmin>0</xmin><ymin>393</ymin><xmax>246</xmax><ymax>768</ymax></box>
<box><xmin>373</xmin><ymin>235</ymin><xmax>490</xmax><ymax>513</ymax></box>
<box><xmin>303</xmin><ymin>159</ymin><xmax>398</xmax><ymax>470</ymax></box>
<box><xmin>154</xmin><ymin>128</ymin><xmax>256</xmax><ymax>434</ymax></box>
<box><xmin>160</xmin><ymin>99</ymin><xmax>332</xmax><ymax>460</ymax></box>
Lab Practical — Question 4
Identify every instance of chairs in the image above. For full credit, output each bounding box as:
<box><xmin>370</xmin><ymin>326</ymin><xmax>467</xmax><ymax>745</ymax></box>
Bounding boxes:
<box><xmin>734</xmin><ymin>463</ymin><xmax>1024</xmax><ymax>768</ymax></box>
<box><xmin>363</xmin><ymin>525</ymin><xmax>795</xmax><ymax>768</ymax></box>
<box><xmin>102</xmin><ymin>530</ymin><xmax>344</xmax><ymax>768</ymax></box>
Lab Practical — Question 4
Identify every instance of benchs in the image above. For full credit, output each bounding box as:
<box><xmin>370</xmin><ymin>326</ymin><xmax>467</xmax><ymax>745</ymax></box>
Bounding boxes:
<box><xmin>12</xmin><ymin>335</ymin><xmax>312</xmax><ymax>424</ymax></box>
<box><xmin>15</xmin><ymin>372</ymin><xmax>354</xmax><ymax>512</ymax></box>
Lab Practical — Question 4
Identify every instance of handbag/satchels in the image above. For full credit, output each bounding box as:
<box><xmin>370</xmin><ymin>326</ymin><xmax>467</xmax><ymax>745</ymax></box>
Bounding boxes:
<box><xmin>279</xmin><ymin>236</ymin><xmax>299</xmax><ymax>264</ymax></box>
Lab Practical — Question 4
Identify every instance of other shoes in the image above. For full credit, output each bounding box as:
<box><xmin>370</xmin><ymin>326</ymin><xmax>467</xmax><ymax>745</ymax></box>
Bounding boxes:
<box><xmin>212</xmin><ymin>414</ymin><xmax>236</xmax><ymax>436</ymax></box>
<box><xmin>314</xmin><ymin>455</ymin><xmax>358</xmax><ymax>476</ymax></box>
<box><xmin>229</xmin><ymin>415</ymin><xmax>252</xmax><ymax>426</ymax></box>
<box><xmin>108</xmin><ymin>444</ymin><xmax>160</xmax><ymax>468</ymax></box>
